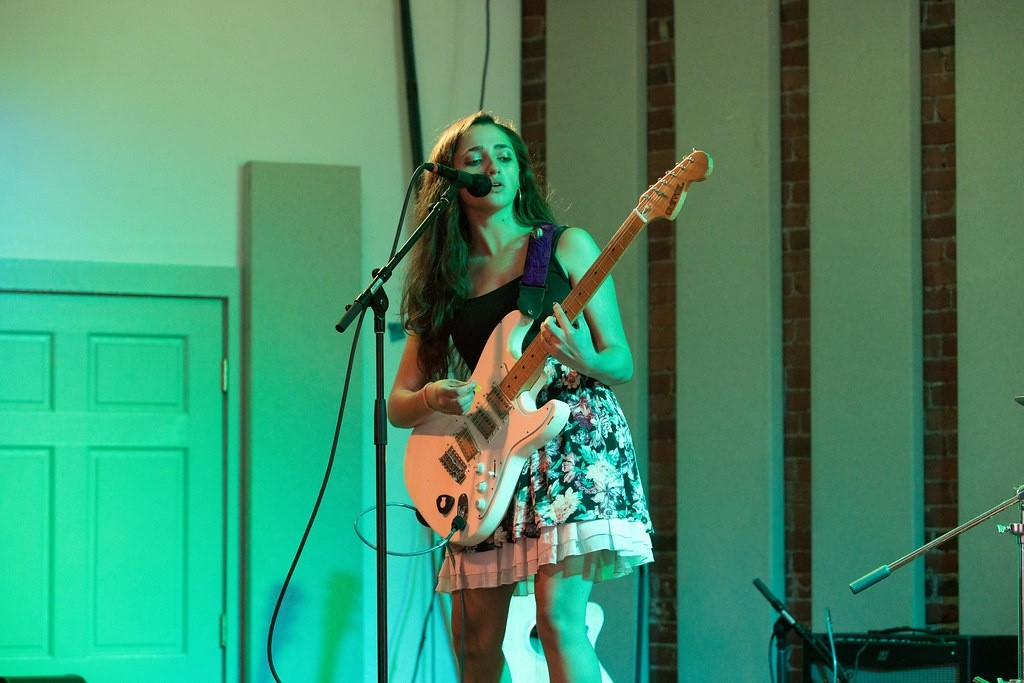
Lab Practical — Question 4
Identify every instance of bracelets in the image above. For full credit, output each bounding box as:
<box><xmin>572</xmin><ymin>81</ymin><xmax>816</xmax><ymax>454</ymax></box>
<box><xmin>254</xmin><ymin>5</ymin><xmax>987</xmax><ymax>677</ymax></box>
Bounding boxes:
<box><xmin>422</xmin><ymin>382</ymin><xmax>435</xmax><ymax>411</ymax></box>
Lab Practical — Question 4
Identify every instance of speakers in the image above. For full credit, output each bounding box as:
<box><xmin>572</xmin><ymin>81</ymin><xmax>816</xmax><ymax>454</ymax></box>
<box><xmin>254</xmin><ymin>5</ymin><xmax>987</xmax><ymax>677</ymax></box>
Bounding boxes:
<box><xmin>803</xmin><ymin>626</ymin><xmax>1019</xmax><ymax>683</ymax></box>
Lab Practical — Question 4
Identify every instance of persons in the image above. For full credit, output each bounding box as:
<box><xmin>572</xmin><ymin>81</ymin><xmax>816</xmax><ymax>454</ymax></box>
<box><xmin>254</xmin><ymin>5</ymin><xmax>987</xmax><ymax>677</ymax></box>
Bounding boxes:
<box><xmin>387</xmin><ymin>112</ymin><xmax>654</xmax><ymax>683</ymax></box>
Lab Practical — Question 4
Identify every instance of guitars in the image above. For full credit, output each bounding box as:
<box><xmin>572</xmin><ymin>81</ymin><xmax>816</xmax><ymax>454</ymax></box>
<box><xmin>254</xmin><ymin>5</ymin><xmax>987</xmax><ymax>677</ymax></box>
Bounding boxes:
<box><xmin>403</xmin><ymin>148</ymin><xmax>715</xmax><ymax>546</ymax></box>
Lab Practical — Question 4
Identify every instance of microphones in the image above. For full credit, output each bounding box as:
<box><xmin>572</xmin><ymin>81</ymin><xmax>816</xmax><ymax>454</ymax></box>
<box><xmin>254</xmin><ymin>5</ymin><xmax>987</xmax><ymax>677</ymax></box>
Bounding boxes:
<box><xmin>422</xmin><ymin>159</ymin><xmax>492</xmax><ymax>198</ymax></box>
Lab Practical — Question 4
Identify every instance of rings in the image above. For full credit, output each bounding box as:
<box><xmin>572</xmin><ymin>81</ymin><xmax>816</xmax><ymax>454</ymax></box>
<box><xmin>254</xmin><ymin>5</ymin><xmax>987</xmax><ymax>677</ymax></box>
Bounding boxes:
<box><xmin>546</xmin><ymin>335</ymin><xmax>551</xmax><ymax>344</ymax></box>
